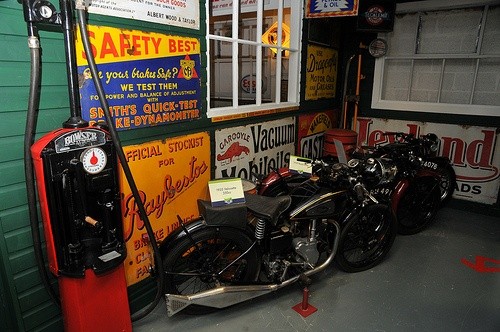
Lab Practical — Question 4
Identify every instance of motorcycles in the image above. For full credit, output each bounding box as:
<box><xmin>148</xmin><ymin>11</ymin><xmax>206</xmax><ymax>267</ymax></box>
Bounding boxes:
<box><xmin>148</xmin><ymin>153</ymin><xmax>407</xmax><ymax>318</ymax></box>
<box><xmin>255</xmin><ymin>128</ymin><xmax>462</xmax><ymax>236</ymax></box>
